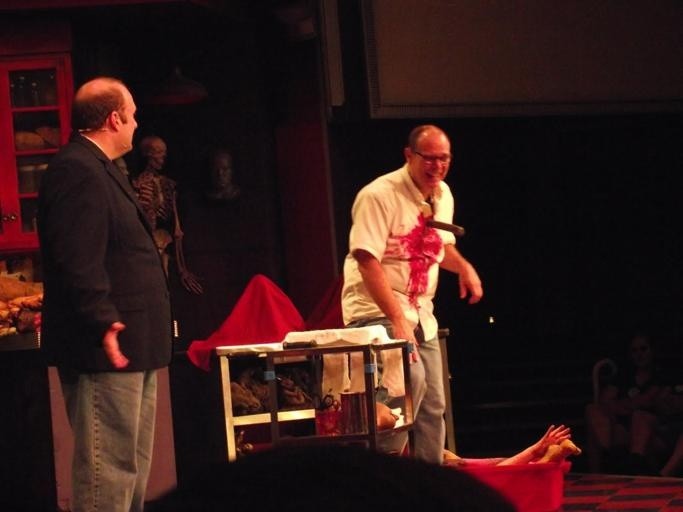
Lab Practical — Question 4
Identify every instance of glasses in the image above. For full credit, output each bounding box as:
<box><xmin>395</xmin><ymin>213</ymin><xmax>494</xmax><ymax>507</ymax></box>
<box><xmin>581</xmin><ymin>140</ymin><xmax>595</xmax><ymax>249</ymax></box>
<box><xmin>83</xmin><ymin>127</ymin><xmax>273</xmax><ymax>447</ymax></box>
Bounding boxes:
<box><xmin>413</xmin><ymin>152</ymin><xmax>452</xmax><ymax>166</ymax></box>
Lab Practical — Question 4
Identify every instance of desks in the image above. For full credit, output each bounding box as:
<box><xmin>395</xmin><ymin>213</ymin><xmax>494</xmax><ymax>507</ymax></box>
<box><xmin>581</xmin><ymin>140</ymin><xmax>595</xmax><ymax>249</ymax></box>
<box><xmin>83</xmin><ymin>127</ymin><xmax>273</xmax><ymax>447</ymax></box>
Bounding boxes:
<box><xmin>0</xmin><ymin>275</ymin><xmax>58</xmax><ymax>512</ymax></box>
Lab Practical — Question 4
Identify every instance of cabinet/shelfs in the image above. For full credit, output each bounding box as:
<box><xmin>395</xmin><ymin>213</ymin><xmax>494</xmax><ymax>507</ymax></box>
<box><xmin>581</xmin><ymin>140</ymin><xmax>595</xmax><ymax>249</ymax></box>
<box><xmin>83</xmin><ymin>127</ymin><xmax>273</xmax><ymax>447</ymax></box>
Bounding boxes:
<box><xmin>216</xmin><ymin>325</ymin><xmax>457</xmax><ymax>464</ymax></box>
<box><xmin>0</xmin><ymin>53</ymin><xmax>73</xmax><ymax>257</ymax></box>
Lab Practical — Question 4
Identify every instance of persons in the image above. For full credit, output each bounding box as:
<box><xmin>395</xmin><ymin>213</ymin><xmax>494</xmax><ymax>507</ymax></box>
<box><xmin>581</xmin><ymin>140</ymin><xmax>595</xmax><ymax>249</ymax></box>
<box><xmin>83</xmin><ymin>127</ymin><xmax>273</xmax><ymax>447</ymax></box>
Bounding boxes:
<box><xmin>37</xmin><ymin>76</ymin><xmax>172</xmax><ymax>512</ymax></box>
<box><xmin>113</xmin><ymin>157</ymin><xmax>129</xmax><ymax>178</ymax></box>
<box><xmin>130</xmin><ymin>135</ymin><xmax>205</xmax><ymax>298</ymax></box>
<box><xmin>340</xmin><ymin>123</ymin><xmax>484</xmax><ymax>465</ymax></box>
<box><xmin>583</xmin><ymin>331</ymin><xmax>683</xmax><ymax>478</ymax></box>
<box><xmin>200</xmin><ymin>153</ymin><xmax>243</xmax><ymax>207</ymax></box>
<box><xmin>497</xmin><ymin>424</ymin><xmax>574</xmax><ymax>466</ymax></box>
